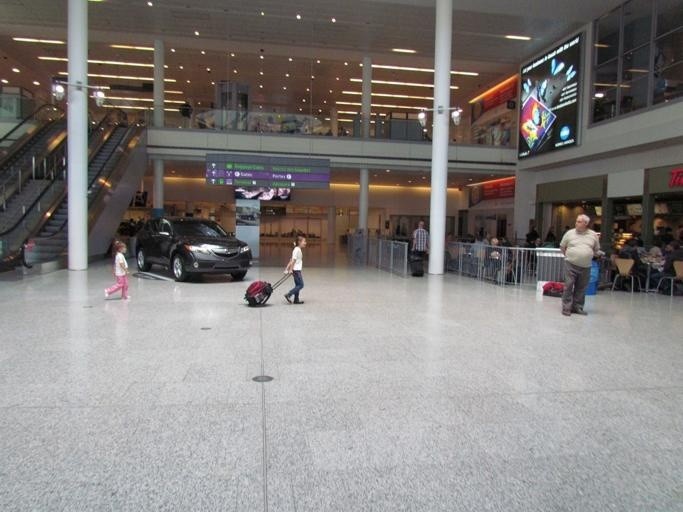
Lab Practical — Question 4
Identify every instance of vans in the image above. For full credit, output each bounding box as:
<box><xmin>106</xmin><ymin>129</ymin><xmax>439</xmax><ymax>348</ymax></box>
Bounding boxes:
<box><xmin>136</xmin><ymin>216</ymin><xmax>252</xmax><ymax>282</ymax></box>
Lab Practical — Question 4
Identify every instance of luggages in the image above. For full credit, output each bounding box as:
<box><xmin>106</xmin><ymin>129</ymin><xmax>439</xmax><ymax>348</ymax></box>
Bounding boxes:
<box><xmin>244</xmin><ymin>281</ymin><xmax>273</xmax><ymax>307</ymax></box>
<box><xmin>410</xmin><ymin>259</ymin><xmax>425</xmax><ymax>277</ymax></box>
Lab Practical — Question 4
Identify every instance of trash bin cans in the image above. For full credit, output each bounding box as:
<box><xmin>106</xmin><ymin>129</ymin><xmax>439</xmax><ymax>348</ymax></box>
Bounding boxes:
<box><xmin>384</xmin><ymin>110</ymin><xmax>424</xmax><ymax>140</ymax></box>
<box><xmin>585</xmin><ymin>258</ymin><xmax>600</xmax><ymax>295</ymax></box>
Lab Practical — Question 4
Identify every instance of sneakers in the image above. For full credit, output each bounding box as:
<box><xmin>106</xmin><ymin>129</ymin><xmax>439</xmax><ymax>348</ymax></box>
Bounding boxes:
<box><xmin>285</xmin><ymin>295</ymin><xmax>303</xmax><ymax>305</ymax></box>
<box><xmin>104</xmin><ymin>289</ymin><xmax>130</xmax><ymax>300</ymax></box>
<box><xmin>561</xmin><ymin>307</ymin><xmax>587</xmax><ymax>317</ymax></box>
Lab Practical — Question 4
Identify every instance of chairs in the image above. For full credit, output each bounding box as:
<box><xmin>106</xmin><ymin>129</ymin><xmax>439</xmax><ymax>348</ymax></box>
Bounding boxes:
<box><xmin>610</xmin><ymin>258</ymin><xmax>643</xmax><ymax>296</ymax></box>
<box><xmin>656</xmin><ymin>259</ymin><xmax>683</xmax><ymax>295</ymax></box>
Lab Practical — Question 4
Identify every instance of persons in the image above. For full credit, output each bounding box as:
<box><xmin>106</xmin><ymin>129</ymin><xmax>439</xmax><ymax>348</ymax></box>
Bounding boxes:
<box><xmin>284</xmin><ymin>236</ymin><xmax>307</xmax><ymax>304</ymax></box>
<box><xmin>104</xmin><ymin>240</ymin><xmax>131</xmax><ymax>299</ymax></box>
<box><xmin>178</xmin><ymin>100</ymin><xmax>193</xmax><ymax>128</ymax></box>
<box><xmin>411</xmin><ymin>220</ymin><xmax>429</xmax><ymax>262</ymax></box>
<box><xmin>446</xmin><ymin>225</ymin><xmax>683</xmax><ymax>294</ymax></box>
<box><xmin>559</xmin><ymin>214</ymin><xmax>600</xmax><ymax>316</ymax></box>
